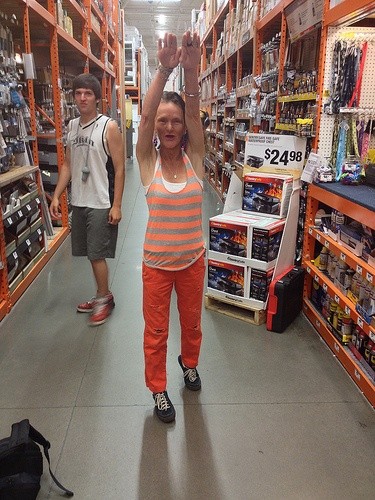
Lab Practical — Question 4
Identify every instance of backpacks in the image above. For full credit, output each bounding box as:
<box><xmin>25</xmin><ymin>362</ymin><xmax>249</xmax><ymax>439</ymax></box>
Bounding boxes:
<box><xmin>0</xmin><ymin>419</ymin><xmax>73</xmax><ymax>500</ymax></box>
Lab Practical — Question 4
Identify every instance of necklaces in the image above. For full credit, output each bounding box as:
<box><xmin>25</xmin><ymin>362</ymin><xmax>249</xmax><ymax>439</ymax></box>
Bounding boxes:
<box><xmin>164</xmin><ymin>155</ymin><xmax>182</xmax><ymax>179</ymax></box>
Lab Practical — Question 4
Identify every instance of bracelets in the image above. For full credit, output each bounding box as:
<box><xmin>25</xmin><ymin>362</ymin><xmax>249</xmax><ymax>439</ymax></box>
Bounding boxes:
<box><xmin>156</xmin><ymin>63</ymin><xmax>173</xmax><ymax>81</ymax></box>
<box><xmin>184</xmin><ymin>84</ymin><xmax>201</xmax><ymax>98</ymax></box>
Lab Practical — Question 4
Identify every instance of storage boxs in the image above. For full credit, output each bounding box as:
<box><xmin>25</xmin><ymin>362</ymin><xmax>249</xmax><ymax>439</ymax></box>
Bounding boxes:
<box><xmin>249</xmin><ymin>217</ymin><xmax>286</xmax><ymax>263</ymax></box>
<box><xmin>247</xmin><ymin>266</ymin><xmax>275</xmax><ymax>302</ymax></box>
<box><xmin>206</xmin><ymin>258</ymin><xmax>246</xmax><ymax>298</ymax></box>
<box><xmin>208</xmin><ymin>214</ymin><xmax>260</xmax><ymax>259</ymax></box>
<box><xmin>242</xmin><ymin>172</ymin><xmax>294</xmax><ymax>218</ymax></box>
<box><xmin>224</xmin><ymin>210</ymin><xmax>272</xmax><ymax>220</ymax></box>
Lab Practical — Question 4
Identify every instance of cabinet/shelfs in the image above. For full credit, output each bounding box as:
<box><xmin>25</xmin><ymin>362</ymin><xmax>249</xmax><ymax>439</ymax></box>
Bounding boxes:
<box><xmin>0</xmin><ymin>0</ymin><xmax>150</xmax><ymax>322</ymax></box>
<box><xmin>181</xmin><ymin>0</ymin><xmax>375</xmax><ymax>412</ymax></box>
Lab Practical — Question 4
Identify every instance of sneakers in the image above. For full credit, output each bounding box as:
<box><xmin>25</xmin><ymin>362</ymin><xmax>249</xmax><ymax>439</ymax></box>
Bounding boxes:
<box><xmin>77</xmin><ymin>290</ymin><xmax>115</xmax><ymax>312</ymax></box>
<box><xmin>153</xmin><ymin>389</ymin><xmax>175</xmax><ymax>423</ymax></box>
<box><xmin>178</xmin><ymin>355</ymin><xmax>201</xmax><ymax>390</ymax></box>
<box><xmin>89</xmin><ymin>294</ymin><xmax>113</xmax><ymax>326</ymax></box>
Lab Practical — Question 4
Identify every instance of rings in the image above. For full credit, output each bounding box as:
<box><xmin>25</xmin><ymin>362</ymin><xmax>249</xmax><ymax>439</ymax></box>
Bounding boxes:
<box><xmin>188</xmin><ymin>43</ymin><xmax>192</xmax><ymax>46</ymax></box>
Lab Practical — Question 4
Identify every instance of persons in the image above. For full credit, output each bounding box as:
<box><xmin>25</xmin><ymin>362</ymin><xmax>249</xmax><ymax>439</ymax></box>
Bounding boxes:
<box><xmin>47</xmin><ymin>72</ymin><xmax>126</xmax><ymax>326</ymax></box>
<box><xmin>133</xmin><ymin>30</ymin><xmax>210</xmax><ymax>424</ymax></box>
